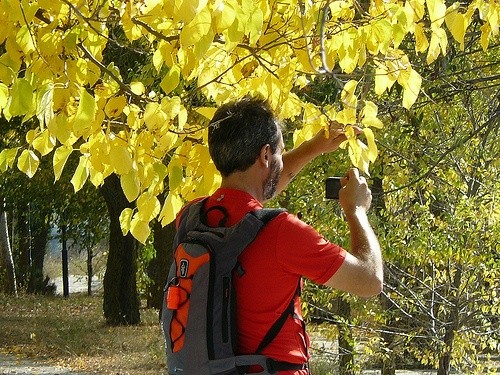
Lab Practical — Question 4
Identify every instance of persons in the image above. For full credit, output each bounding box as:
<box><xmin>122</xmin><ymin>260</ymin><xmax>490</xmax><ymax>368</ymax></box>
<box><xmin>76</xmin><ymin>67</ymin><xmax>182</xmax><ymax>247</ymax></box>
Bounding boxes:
<box><xmin>176</xmin><ymin>92</ymin><xmax>384</xmax><ymax>375</ymax></box>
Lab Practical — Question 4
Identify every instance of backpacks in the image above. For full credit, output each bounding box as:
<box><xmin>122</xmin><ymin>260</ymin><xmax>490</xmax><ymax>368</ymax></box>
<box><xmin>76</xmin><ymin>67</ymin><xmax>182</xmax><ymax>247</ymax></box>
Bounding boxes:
<box><xmin>162</xmin><ymin>197</ymin><xmax>310</xmax><ymax>375</ymax></box>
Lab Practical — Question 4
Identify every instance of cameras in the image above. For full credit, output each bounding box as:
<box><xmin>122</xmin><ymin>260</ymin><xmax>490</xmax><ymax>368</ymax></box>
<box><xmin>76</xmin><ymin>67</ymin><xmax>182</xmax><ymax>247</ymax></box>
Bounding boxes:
<box><xmin>326</xmin><ymin>177</ymin><xmax>348</xmax><ymax>199</ymax></box>
